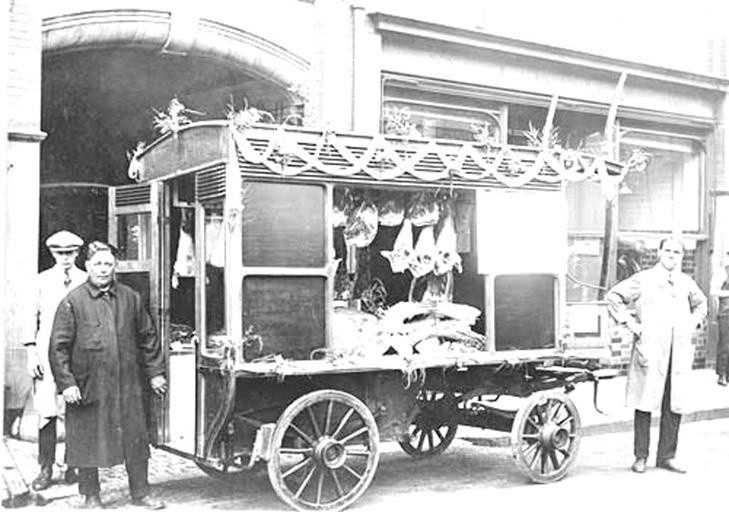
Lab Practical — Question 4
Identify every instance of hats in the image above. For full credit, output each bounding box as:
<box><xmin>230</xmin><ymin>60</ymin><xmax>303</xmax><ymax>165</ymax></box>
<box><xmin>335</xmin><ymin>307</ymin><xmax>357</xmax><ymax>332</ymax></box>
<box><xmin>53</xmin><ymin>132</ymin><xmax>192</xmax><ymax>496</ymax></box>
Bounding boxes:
<box><xmin>46</xmin><ymin>231</ymin><xmax>84</xmax><ymax>252</ymax></box>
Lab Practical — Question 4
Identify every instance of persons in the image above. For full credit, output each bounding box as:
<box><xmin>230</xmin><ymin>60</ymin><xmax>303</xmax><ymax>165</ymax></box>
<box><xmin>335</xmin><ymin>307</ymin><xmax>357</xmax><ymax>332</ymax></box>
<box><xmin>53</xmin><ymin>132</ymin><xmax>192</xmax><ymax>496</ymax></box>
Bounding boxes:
<box><xmin>49</xmin><ymin>241</ymin><xmax>168</xmax><ymax>510</ymax></box>
<box><xmin>21</xmin><ymin>230</ymin><xmax>90</xmax><ymax>492</ymax></box>
<box><xmin>604</xmin><ymin>238</ymin><xmax>707</xmax><ymax>475</ymax></box>
<box><xmin>710</xmin><ymin>252</ymin><xmax>729</xmax><ymax>386</ymax></box>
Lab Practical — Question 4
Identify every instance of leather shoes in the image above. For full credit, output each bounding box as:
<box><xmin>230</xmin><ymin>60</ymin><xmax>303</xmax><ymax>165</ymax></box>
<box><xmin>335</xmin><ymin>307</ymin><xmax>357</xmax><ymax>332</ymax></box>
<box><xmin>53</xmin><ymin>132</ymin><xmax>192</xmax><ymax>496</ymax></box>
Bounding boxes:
<box><xmin>656</xmin><ymin>460</ymin><xmax>686</xmax><ymax>473</ymax></box>
<box><xmin>132</xmin><ymin>494</ymin><xmax>164</xmax><ymax>510</ymax></box>
<box><xmin>60</xmin><ymin>469</ymin><xmax>79</xmax><ymax>484</ymax></box>
<box><xmin>33</xmin><ymin>470</ymin><xmax>51</xmax><ymax>490</ymax></box>
<box><xmin>633</xmin><ymin>457</ymin><xmax>647</xmax><ymax>472</ymax></box>
<box><xmin>85</xmin><ymin>496</ymin><xmax>104</xmax><ymax>509</ymax></box>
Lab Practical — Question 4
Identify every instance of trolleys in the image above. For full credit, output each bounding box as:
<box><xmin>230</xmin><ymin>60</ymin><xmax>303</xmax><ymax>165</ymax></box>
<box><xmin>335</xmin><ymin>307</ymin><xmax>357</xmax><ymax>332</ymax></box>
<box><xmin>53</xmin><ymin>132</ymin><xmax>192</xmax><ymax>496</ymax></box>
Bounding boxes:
<box><xmin>129</xmin><ymin>120</ymin><xmax>647</xmax><ymax>512</ymax></box>
